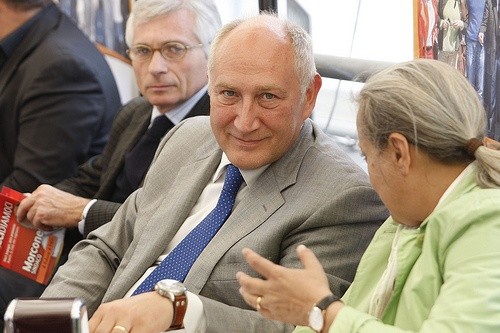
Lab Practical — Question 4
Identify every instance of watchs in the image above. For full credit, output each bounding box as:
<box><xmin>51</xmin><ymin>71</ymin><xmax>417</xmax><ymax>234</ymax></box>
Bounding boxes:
<box><xmin>308</xmin><ymin>295</ymin><xmax>339</xmax><ymax>333</ymax></box>
<box><xmin>152</xmin><ymin>279</ymin><xmax>188</xmax><ymax>330</ymax></box>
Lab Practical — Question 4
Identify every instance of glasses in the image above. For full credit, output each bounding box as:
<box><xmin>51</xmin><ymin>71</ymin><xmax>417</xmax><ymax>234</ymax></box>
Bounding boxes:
<box><xmin>126</xmin><ymin>40</ymin><xmax>203</xmax><ymax>63</ymax></box>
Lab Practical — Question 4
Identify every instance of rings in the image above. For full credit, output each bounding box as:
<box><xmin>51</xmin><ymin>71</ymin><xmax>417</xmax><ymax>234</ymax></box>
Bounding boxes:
<box><xmin>257</xmin><ymin>297</ymin><xmax>261</xmax><ymax>310</ymax></box>
<box><xmin>113</xmin><ymin>326</ymin><xmax>127</xmax><ymax>333</ymax></box>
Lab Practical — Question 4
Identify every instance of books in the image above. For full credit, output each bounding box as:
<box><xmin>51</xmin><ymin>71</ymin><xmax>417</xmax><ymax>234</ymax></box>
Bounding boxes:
<box><xmin>0</xmin><ymin>185</ymin><xmax>65</xmax><ymax>285</ymax></box>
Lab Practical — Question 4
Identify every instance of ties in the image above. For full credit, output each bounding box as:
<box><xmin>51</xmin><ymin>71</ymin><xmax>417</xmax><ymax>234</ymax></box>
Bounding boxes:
<box><xmin>110</xmin><ymin>114</ymin><xmax>173</xmax><ymax>204</ymax></box>
<box><xmin>131</xmin><ymin>164</ymin><xmax>244</xmax><ymax>296</ymax></box>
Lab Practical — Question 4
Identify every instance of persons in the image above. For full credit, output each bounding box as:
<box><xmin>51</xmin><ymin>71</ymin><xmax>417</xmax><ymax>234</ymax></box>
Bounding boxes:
<box><xmin>40</xmin><ymin>15</ymin><xmax>389</xmax><ymax>333</ymax></box>
<box><xmin>17</xmin><ymin>1</ymin><xmax>223</xmax><ymax>238</ymax></box>
<box><xmin>236</xmin><ymin>59</ymin><xmax>500</xmax><ymax>333</ymax></box>
<box><xmin>419</xmin><ymin>0</ymin><xmax>500</xmax><ymax>139</ymax></box>
<box><xmin>0</xmin><ymin>0</ymin><xmax>122</xmax><ymax>196</ymax></box>
